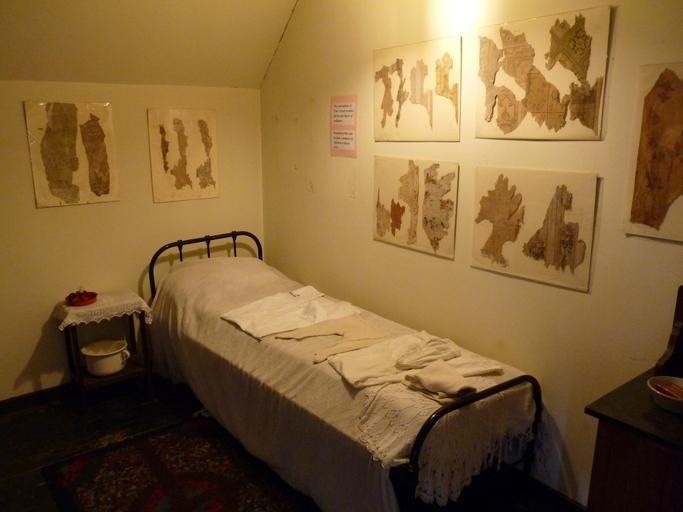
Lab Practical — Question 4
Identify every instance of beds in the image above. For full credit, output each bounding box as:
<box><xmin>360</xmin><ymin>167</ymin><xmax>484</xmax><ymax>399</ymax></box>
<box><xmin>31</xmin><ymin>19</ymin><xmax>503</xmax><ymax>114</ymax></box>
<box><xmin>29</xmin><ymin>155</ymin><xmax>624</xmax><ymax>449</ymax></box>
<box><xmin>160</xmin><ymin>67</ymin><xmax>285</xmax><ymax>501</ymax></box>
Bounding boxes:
<box><xmin>149</xmin><ymin>230</ymin><xmax>543</xmax><ymax>512</ymax></box>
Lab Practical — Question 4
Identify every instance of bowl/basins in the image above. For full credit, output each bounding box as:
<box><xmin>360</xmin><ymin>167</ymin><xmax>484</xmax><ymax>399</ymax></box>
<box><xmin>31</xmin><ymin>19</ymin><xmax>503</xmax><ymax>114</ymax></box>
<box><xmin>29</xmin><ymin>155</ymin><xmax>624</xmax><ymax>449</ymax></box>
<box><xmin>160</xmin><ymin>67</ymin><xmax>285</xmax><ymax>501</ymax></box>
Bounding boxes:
<box><xmin>646</xmin><ymin>376</ymin><xmax>683</xmax><ymax>412</ymax></box>
<box><xmin>81</xmin><ymin>340</ymin><xmax>129</xmax><ymax>376</ymax></box>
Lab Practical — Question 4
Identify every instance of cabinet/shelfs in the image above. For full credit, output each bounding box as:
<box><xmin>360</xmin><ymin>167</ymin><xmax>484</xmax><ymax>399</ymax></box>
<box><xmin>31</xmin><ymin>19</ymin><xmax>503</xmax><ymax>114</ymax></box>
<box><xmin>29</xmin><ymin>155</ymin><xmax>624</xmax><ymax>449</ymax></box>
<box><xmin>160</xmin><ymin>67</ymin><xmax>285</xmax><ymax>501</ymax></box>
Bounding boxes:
<box><xmin>59</xmin><ymin>289</ymin><xmax>156</xmax><ymax>431</ymax></box>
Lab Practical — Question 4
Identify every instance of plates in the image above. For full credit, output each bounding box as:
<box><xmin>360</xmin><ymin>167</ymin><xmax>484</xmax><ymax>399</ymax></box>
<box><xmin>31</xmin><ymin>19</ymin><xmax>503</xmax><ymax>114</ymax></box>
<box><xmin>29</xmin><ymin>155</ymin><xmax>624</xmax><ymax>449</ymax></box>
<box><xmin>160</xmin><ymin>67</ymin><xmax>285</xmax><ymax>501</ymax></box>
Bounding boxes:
<box><xmin>65</xmin><ymin>292</ymin><xmax>97</xmax><ymax>306</ymax></box>
